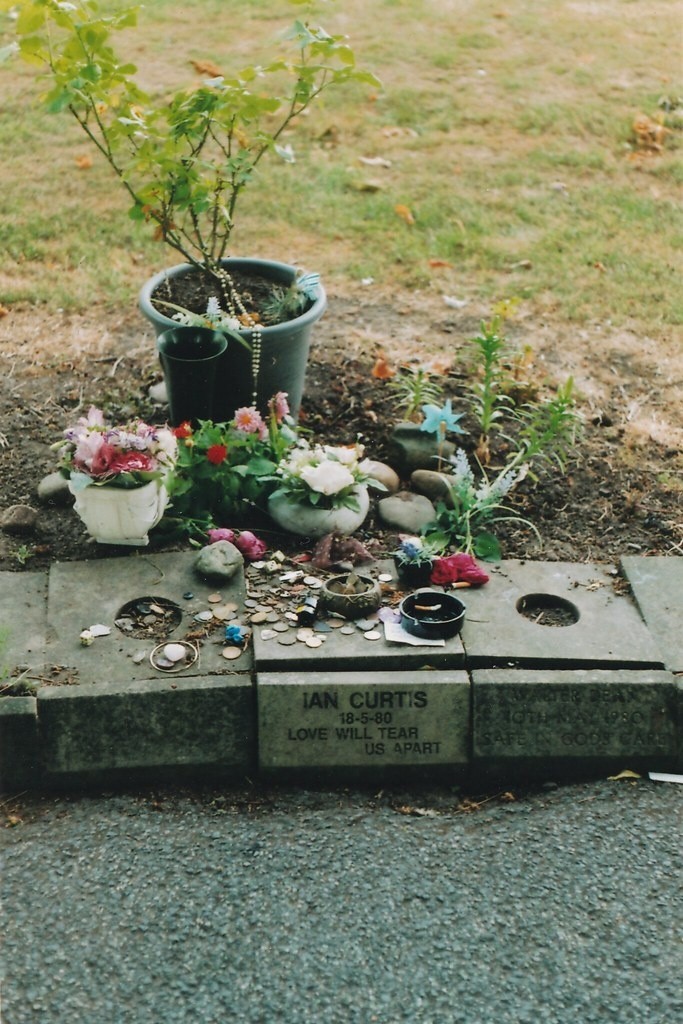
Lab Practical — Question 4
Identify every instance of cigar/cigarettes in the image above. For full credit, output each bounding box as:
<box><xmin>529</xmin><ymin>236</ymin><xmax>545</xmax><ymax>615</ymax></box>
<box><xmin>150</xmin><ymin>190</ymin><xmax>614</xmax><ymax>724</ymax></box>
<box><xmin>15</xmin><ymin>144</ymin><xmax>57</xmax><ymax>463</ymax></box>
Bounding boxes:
<box><xmin>451</xmin><ymin>581</ymin><xmax>471</xmax><ymax>589</ymax></box>
<box><xmin>414</xmin><ymin>604</ymin><xmax>442</xmax><ymax>611</ymax></box>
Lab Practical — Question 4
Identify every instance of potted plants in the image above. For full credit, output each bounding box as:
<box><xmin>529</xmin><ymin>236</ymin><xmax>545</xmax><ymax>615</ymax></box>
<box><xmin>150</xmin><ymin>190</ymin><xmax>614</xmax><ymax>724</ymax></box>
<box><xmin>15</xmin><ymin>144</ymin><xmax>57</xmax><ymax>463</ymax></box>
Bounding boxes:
<box><xmin>0</xmin><ymin>0</ymin><xmax>363</xmax><ymax>427</ymax></box>
<box><xmin>320</xmin><ymin>574</ymin><xmax>381</xmax><ymax>617</ymax></box>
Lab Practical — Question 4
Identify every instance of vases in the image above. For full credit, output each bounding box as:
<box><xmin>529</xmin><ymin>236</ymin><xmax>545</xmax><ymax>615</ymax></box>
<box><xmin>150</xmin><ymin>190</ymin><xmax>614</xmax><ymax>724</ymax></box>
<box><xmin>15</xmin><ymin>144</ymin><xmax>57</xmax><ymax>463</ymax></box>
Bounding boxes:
<box><xmin>68</xmin><ymin>474</ymin><xmax>169</xmax><ymax>546</ymax></box>
<box><xmin>157</xmin><ymin>328</ymin><xmax>227</xmax><ymax>429</ymax></box>
<box><xmin>267</xmin><ymin>488</ymin><xmax>369</xmax><ymax>537</ymax></box>
<box><xmin>393</xmin><ymin>549</ymin><xmax>433</xmax><ymax>588</ymax></box>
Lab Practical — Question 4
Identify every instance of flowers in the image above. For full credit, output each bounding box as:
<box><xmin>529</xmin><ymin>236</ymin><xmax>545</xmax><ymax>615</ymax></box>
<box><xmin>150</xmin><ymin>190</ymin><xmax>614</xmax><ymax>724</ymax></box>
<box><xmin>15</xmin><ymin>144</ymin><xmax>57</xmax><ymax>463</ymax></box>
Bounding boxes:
<box><xmin>49</xmin><ymin>404</ymin><xmax>389</xmax><ymax>512</ymax></box>
<box><xmin>389</xmin><ymin>536</ymin><xmax>438</xmax><ymax>569</ymax></box>
<box><xmin>148</xmin><ymin>295</ymin><xmax>254</xmax><ymax>353</ymax></box>
